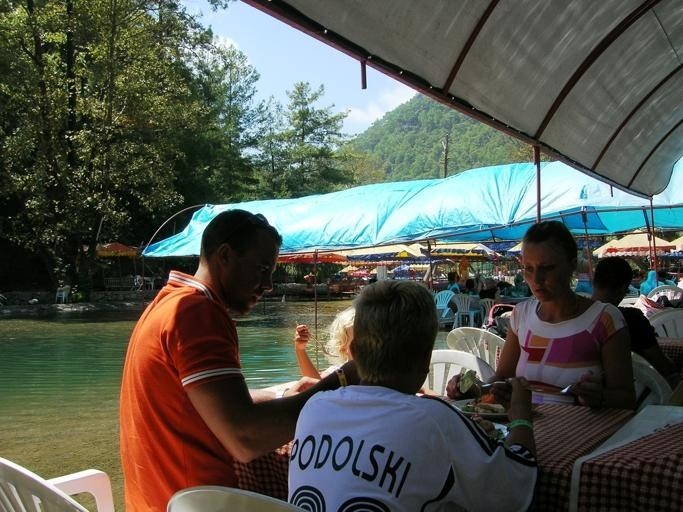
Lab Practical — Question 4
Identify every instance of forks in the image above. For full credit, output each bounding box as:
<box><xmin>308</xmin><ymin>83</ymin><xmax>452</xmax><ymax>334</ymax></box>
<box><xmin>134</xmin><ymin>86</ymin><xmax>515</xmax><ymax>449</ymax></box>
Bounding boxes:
<box><xmin>471</xmin><ymin>379</ymin><xmax>511</xmax><ymax>388</ymax></box>
<box><xmin>556</xmin><ymin>369</ymin><xmax>608</xmax><ymax>395</ymax></box>
<box><xmin>296</xmin><ymin>321</ymin><xmax>337</xmax><ymax>358</ymax></box>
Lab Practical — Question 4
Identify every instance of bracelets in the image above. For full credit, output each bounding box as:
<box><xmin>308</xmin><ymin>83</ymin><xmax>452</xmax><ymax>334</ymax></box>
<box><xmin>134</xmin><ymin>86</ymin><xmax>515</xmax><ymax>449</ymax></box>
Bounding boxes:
<box><xmin>506</xmin><ymin>418</ymin><xmax>534</xmax><ymax>430</ymax></box>
<box><xmin>335</xmin><ymin>368</ymin><xmax>346</xmax><ymax>388</ymax></box>
<box><xmin>276</xmin><ymin>384</ymin><xmax>287</xmax><ymax>402</ymax></box>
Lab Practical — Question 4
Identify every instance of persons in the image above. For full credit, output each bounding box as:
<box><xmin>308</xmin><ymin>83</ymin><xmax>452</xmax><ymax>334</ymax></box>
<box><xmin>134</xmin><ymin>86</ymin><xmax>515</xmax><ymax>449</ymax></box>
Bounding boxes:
<box><xmin>446</xmin><ymin>220</ymin><xmax>635</xmax><ymax>410</ymax></box>
<box><xmin>286</xmin><ymin>280</ymin><xmax>539</xmax><ymax>512</ymax></box>
<box><xmin>292</xmin><ymin>307</ymin><xmax>357</xmax><ymax>390</ymax></box>
<box><xmin>589</xmin><ymin>256</ymin><xmax>680</xmax><ymax>388</ymax></box>
<box><xmin>448</xmin><ymin>272</ymin><xmax>515</xmax><ymax>302</ymax></box>
<box><xmin>120</xmin><ymin>207</ymin><xmax>358</xmax><ymax>512</ymax></box>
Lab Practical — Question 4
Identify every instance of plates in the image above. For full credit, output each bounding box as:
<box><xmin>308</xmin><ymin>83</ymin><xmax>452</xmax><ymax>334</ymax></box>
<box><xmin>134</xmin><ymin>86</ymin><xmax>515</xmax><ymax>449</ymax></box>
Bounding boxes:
<box><xmin>445</xmin><ymin>397</ymin><xmax>532</xmax><ymax>417</ymax></box>
<box><xmin>493</xmin><ymin>422</ymin><xmax>511</xmax><ymax>443</ymax></box>
